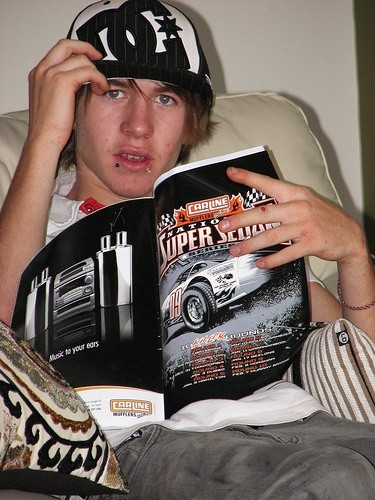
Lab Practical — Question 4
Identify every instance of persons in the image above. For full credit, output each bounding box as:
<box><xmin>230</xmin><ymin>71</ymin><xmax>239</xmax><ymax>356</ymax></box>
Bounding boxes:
<box><xmin>2</xmin><ymin>1</ymin><xmax>375</xmax><ymax>500</ymax></box>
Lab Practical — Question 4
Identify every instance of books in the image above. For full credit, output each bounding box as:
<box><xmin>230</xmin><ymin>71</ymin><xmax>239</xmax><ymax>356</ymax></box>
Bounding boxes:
<box><xmin>8</xmin><ymin>143</ymin><xmax>312</xmax><ymax>427</ymax></box>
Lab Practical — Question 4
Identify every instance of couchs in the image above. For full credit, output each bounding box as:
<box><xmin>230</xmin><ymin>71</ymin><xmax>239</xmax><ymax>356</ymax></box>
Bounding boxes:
<box><xmin>1</xmin><ymin>93</ymin><xmax>343</xmax><ymax>500</ymax></box>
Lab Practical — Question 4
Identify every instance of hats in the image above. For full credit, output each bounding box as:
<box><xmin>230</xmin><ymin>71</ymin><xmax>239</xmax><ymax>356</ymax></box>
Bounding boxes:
<box><xmin>66</xmin><ymin>1</ymin><xmax>213</xmax><ymax>107</ymax></box>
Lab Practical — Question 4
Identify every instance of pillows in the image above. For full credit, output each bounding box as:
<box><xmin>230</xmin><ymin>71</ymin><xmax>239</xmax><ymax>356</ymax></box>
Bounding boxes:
<box><xmin>0</xmin><ymin>318</ymin><xmax>131</xmax><ymax>493</ymax></box>
<box><xmin>280</xmin><ymin>317</ymin><xmax>374</xmax><ymax>423</ymax></box>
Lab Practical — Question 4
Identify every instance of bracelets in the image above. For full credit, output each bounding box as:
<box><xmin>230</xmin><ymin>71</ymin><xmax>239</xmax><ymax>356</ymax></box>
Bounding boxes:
<box><xmin>337</xmin><ymin>254</ymin><xmax>375</xmax><ymax>311</ymax></box>
